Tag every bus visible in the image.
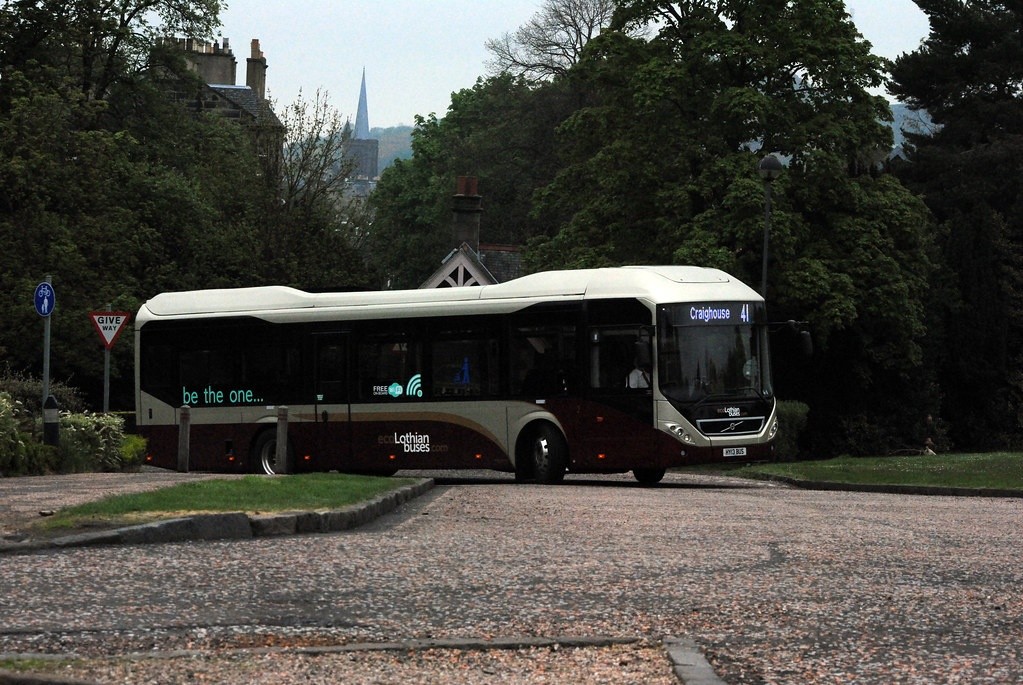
[133,263,782,486]
